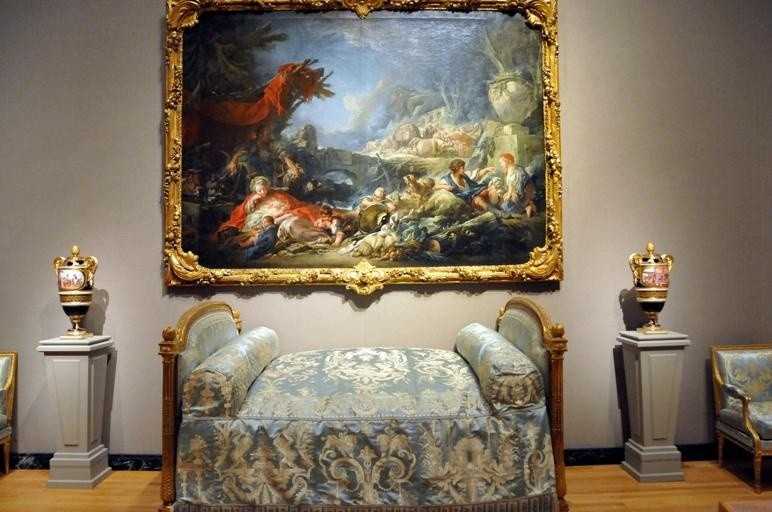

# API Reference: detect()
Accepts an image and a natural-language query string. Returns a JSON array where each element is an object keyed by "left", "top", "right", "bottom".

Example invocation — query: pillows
[
  {"left": 181, "top": 326, "right": 280, "bottom": 419},
  {"left": 455, "top": 321, "right": 546, "bottom": 414}
]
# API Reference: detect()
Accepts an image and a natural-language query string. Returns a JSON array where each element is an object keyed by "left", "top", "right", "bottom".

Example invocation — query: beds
[{"left": 159, "top": 297, "right": 569, "bottom": 512}]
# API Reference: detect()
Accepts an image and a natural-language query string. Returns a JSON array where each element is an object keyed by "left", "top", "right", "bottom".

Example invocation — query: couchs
[
  {"left": 0, "top": 352, "right": 18, "bottom": 475},
  {"left": 709, "top": 344, "right": 772, "bottom": 494}
]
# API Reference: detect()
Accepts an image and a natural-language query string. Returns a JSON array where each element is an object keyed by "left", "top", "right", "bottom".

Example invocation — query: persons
[
  {"left": 238, "top": 216, "right": 277, "bottom": 260},
  {"left": 354, "top": 187, "right": 396, "bottom": 215},
  {"left": 215, "top": 176, "right": 351, "bottom": 247},
  {"left": 314, "top": 204, "right": 345, "bottom": 247},
  {"left": 499, "top": 153, "right": 537, "bottom": 218},
  {"left": 432, "top": 159, "right": 512, "bottom": 219}
]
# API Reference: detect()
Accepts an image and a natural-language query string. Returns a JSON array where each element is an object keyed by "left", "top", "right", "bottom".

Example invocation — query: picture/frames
[{"left": 162, "top": 0, "right": 564, "bottom": 296}]
